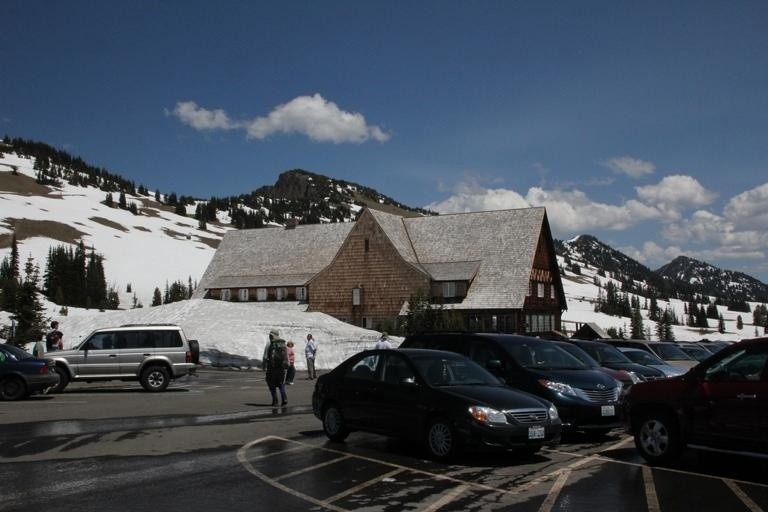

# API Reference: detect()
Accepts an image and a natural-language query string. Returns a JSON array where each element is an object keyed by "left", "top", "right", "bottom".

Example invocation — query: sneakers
[
  {"left": 305, "top": 377, "right": 313, "bottom": 380},
  {"left": 271, "top": 400, "right": 278, "bottom": 406},
  {"left": 289, "top": 382, "right": 294, "bottom": 385},
  {"left": 281, "top": 399, "right": 288, "bottom": 407}
]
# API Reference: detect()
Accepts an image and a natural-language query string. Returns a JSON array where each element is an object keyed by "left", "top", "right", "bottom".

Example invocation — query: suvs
[
  {"left": 616, "top": 335, "right": 768, "bottom": 466},
  {"left": 34, "top": 323, "right": 203, "bottom": 395}
]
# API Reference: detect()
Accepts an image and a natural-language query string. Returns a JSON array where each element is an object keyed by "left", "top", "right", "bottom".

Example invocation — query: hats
[
  {"left": 382, "top": 332, "right": 388, "bottom": 339},
  {"left": 264, "top": 328, "right": 282, "bottom": 340}
]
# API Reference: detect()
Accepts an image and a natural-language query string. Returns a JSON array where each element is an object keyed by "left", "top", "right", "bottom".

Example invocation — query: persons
[
  {"left": 46, "top": 321, "right": 64, "bottom": 352},
  {"left": 373, "top": 331, "right": 391, "bottom": 363},
  {"left": 286, "top": 341, "right": 296, "bottom": 385},
  {"left": 33, "top": 334, "right": 49, "bottom": 395},
  {"left": 305, "top": 334, "right": 317, "bottom": 380},
  {"left": 261, "top": 329, "right": 288, "bottom": 405}
]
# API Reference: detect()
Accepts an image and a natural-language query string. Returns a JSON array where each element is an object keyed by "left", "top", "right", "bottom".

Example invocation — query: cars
[
  {"left": 0, "top": 342, "right": 63, "bottom": 402},
  {"left": 519, "top": 326, "right": 736, "bottom": 408},
  {"left": 311, "top": 344, "right": 564, "bottom": 467}
]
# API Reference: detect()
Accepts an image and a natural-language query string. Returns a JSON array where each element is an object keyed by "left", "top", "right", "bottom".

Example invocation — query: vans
[{"left": 394, "top": 327, "right": 625, "bottom": 446}]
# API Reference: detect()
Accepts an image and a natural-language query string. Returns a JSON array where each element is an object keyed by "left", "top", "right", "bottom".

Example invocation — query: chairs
[{"left": 355, "top": 365, "right": 370, "bottom": 379}]
